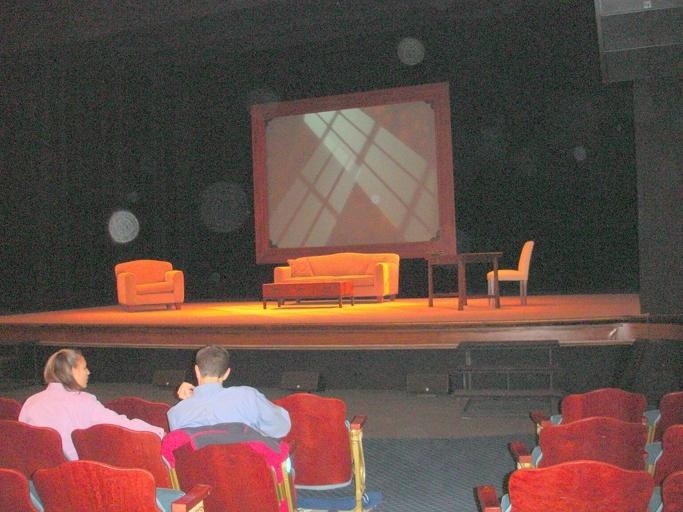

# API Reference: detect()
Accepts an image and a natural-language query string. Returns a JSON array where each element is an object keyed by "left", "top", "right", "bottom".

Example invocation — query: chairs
[
  {"left": 487, "top": 241, "right": 535, "bottom": 308},
  {"left": 115, "top": 260, "right": 185, "bottom": 312},
  {"left": 477, "top": 387, "right": 683, "bottom": 512}
]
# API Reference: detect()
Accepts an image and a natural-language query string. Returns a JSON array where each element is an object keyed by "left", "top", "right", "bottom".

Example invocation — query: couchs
[{"left": 274, "top": 252, "right": 400, "bottom": 304}]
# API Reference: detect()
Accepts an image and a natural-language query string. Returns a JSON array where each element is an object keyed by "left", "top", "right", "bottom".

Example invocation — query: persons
[
  {"left": 18, "top": 349, "right": 167, "bottom": 461},
  {"left": 166, "top": 344, "right": 291, "bottom": 439}
]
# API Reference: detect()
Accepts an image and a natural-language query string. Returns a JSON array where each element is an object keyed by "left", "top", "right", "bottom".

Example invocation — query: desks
[{"left": 428, "top": 252, "right": 502, "bottom": 310}]
